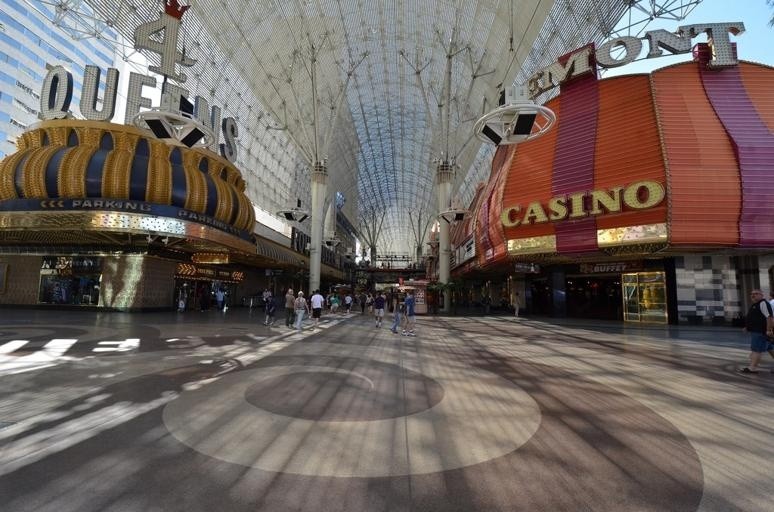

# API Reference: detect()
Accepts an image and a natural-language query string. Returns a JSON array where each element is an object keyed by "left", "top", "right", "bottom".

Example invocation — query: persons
[
  {"left": 740, "top": 289, "right": 774, "bottom": 374},
  {"left": 513, "top": 291, "right": 521, "bottom": 317},
  {"left": 198, "top": 287, "right": 225, "bottom": 312},
  {"left": 178, "top": 283, "right": 189, "bottom": 312},
  {"left": 262, "top": 287, "right": 416, "bottom": 336}
]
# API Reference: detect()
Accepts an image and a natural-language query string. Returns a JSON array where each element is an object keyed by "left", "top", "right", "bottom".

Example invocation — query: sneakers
[{"left": 372, "top": 324, "right": 416, "bottom": 337}]
